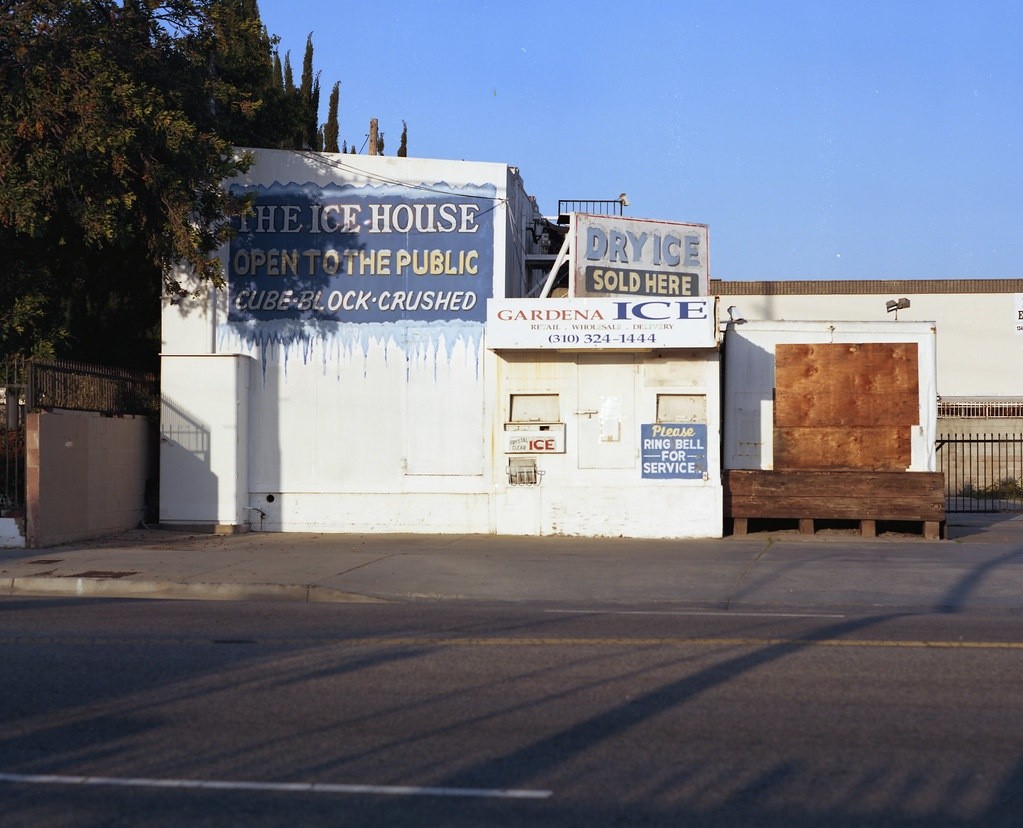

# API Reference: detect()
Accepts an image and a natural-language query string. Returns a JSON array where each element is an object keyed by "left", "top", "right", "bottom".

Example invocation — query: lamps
[
  {"left": 885, "top": 298, "right": 911, "bottom": 320},
  {"left": 618, "top": 193, "right": 631, "bottom": 207}
]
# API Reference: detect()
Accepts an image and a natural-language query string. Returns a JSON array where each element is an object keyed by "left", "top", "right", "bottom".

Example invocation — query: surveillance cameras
[
  {"left": 728, "top": 306, "right": 743, "bottom": 322},
  {"left": 620, "top": 193, "right": 631, "bottom": 207}
]
